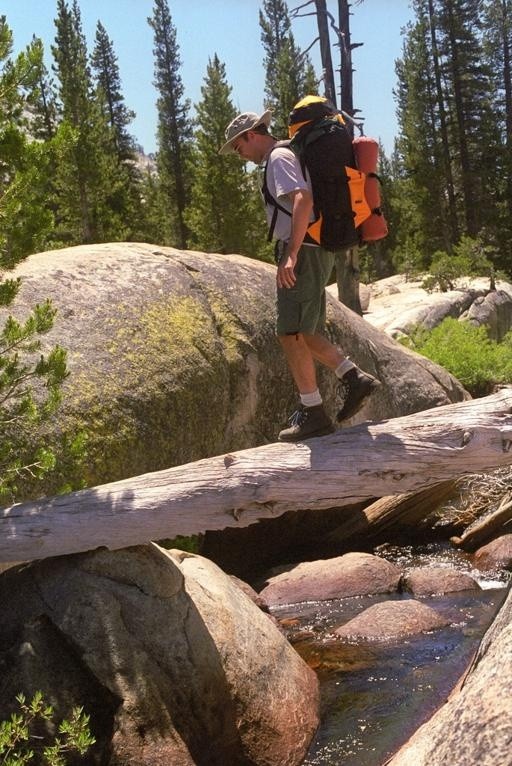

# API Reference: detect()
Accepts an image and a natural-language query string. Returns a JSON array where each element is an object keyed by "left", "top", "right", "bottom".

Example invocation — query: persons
[{"left": 218, "top": 95, "right": 389, "bottom": 442}]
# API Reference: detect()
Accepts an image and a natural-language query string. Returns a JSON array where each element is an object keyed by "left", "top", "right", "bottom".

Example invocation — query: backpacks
[{"left": 285, "top": 94, "right": 371, "bottom": 252}]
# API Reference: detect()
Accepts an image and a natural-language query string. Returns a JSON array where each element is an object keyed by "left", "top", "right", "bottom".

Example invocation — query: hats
[{"left": 218, "top": 110, "right": 272, "bottom": 157}]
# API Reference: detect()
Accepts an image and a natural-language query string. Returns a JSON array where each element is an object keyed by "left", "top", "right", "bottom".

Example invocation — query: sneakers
[
  {"left": 334, "top": 367, "right": 381, "bottom": 424},
  {"left": 276, "top": 402, "right": 336, "bottom": 443}
]
